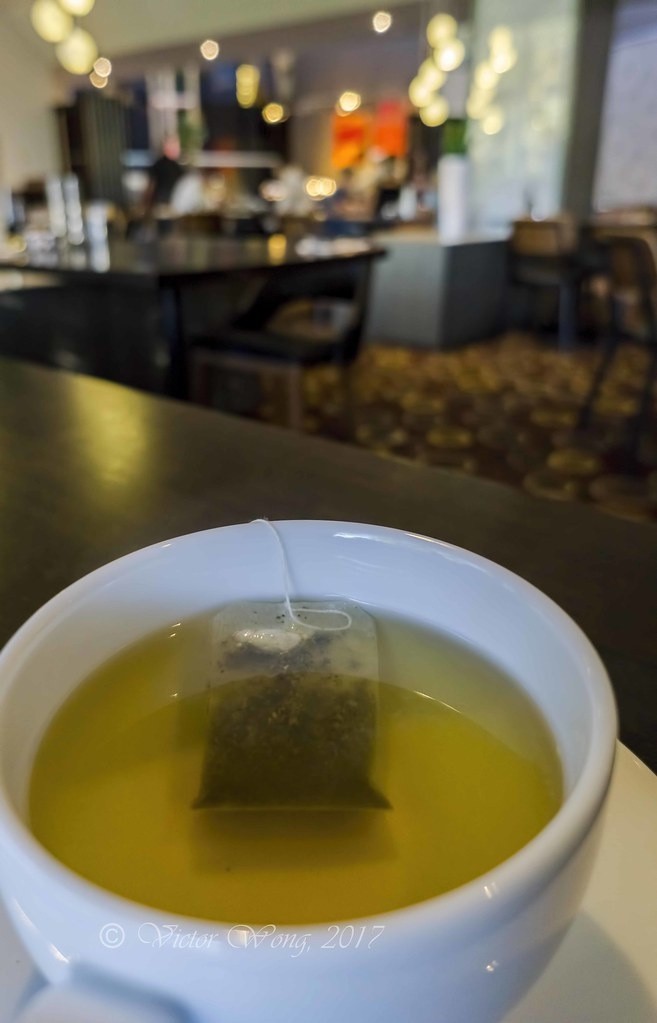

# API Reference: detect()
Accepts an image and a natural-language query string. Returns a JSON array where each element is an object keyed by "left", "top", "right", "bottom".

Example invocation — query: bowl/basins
[{"left": 0, "top": 516, "right": 619, "bottom": 1022}]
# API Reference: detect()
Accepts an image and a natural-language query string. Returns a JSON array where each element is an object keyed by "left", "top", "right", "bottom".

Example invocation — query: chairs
[{"left": 570, "top": 227, "right": 657, "bottom": 488}]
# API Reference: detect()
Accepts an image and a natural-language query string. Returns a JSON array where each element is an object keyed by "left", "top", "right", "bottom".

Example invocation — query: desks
[{"left": 366, "top": 217, "right": 513, "bottom": 350}]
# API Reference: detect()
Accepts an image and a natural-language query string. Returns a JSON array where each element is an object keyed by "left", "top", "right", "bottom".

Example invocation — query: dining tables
[
  {"left": 0, "top": 221, "right": 385, "bottom": 438},
  {"left": 0, "top": 353, "right": 657, "bottom": 1020}
]
